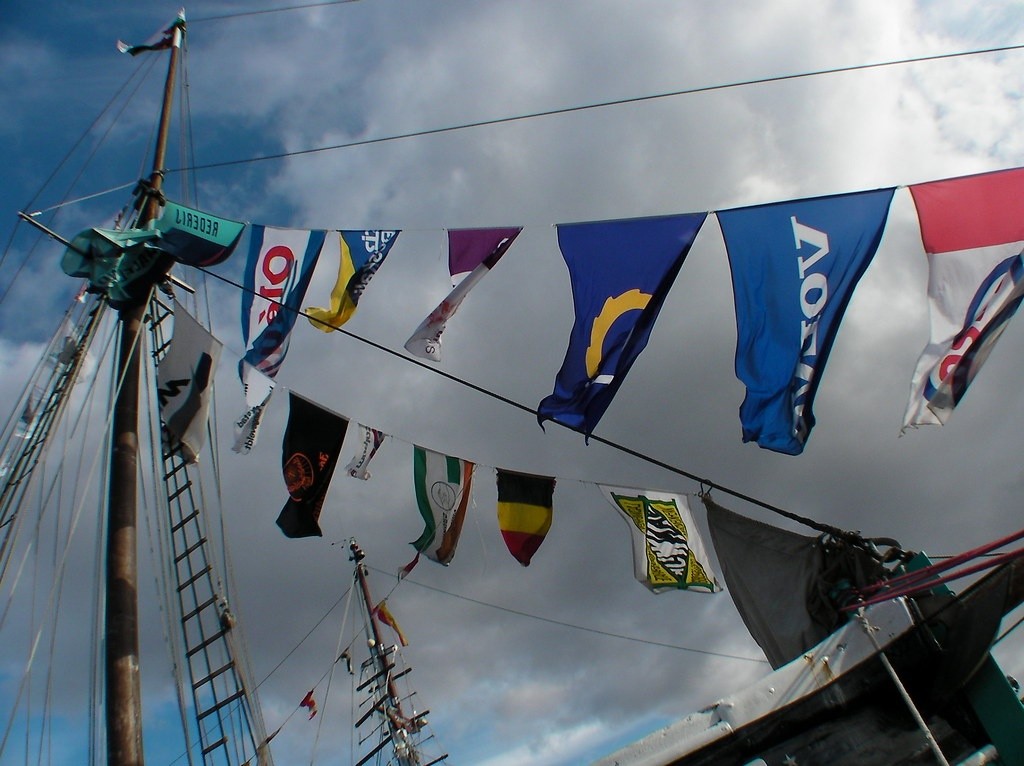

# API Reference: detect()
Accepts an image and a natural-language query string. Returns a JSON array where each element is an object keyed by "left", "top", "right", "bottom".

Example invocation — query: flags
[
  {"left": 408, "top": 445, "right": 474, "bottom": 567},
  {"left": 900, "top": 167, "right": 1024, "bottom": 433},
  {"left": 599, "top": 484, "right": 722, "bottom": 595},
  {"left": 276, "top": 392, "right": 348, "bottom": 538},
  {"left": 405, "top": 227, "right": 524, "bottom": 361},
  {"left": 345, "top": 425, "right": 385, "bottom": 480},
  {"left": 378, "top": 604, "right": 408, "bottom": 646},
  {"left": 240, "top": 225, "right": 325, "bottom": 376},
  {"left": 232, "top": 360, "right": 277, "bottom": 454},
  {"left": 716, "top": 187, "right": 896, "bottom": 456},
  {"left": 61, "top": 202, "right": 246, "bottom": 310},
  {"left": 495, "top": 468, "right": 557, "bottom": 569},
  {"left": 301, "top": 693, "right": 319, "bottom": 719},
  {"left": 305, "top": 230, "right": 401, "bottom": 333},
  {"left": 158, "top": 299, "right": 221, "bottom": 460},
  {"left": 536, "top": 211, "right": 707, "bottom": 446}
]
